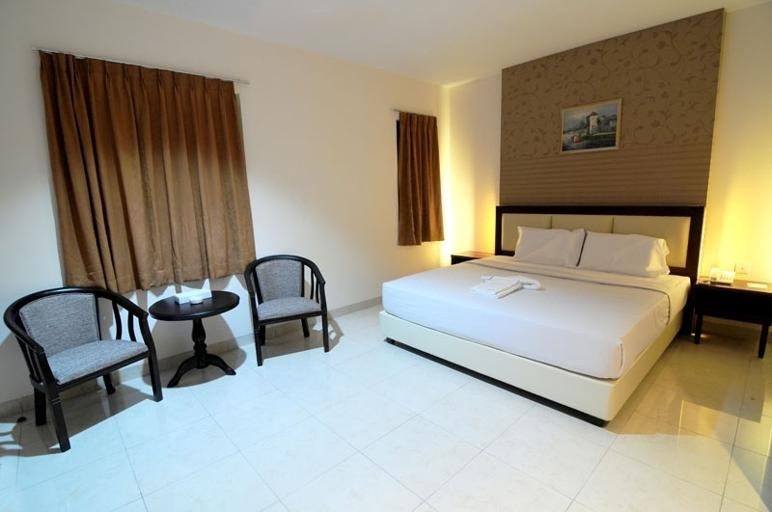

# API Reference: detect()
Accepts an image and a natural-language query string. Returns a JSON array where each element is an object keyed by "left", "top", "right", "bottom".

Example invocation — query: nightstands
[
  {"left": 451, "top": 251, "right": 494, "bottom": 265},
  {"left": 694, "top": 278, "right": 772, "bottom": 359}
]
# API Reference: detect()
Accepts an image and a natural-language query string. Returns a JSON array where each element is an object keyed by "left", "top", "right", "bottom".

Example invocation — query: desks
[{"left": 148, "top": 290, "right": 240, "bottom": 388}]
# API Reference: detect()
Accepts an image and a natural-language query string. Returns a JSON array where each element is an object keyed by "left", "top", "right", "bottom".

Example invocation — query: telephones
[{"left": 710, "top": 268, "right": 736, "bottom": 285}]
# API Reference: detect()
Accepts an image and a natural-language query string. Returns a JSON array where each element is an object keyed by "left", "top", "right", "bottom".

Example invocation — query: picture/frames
[{"left": 559, "top": 96, "right": 622, "bottom": 156}]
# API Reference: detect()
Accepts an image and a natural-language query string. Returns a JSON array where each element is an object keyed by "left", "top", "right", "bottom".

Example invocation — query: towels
[
  {"left": 480, "top": 274, "right": 542, "bottom": 290},
  {"left": 471, "top": 278, "right": 523, "bottom": 300}
]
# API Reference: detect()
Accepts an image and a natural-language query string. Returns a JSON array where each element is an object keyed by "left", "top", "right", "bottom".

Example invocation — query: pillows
[
  {"left": 577, "top": 230, "right": 671, "bottom": 278},
  {"left": 512, "top": 226, "right": 586, "bottom": 268}
]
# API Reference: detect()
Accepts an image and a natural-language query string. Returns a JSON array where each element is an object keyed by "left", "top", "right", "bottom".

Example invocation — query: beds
[{"left": 378, "top": 205, "right": 704, "bottom": 427}]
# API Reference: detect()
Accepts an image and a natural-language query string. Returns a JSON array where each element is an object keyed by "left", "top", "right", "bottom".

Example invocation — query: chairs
[
  {"left": 3, "top": 287, "right": 163, "bottom": 452},
  {"left": 244, "top": 254, "right": 329, "bottom": 366}
]
